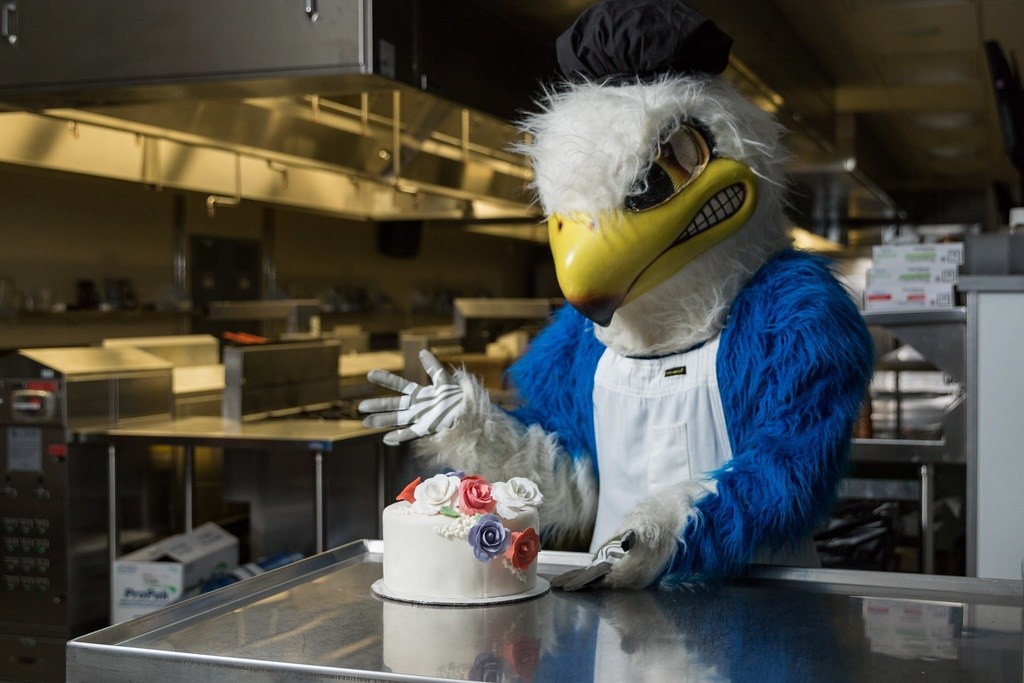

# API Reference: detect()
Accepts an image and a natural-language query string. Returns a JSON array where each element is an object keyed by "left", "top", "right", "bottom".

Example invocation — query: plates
[{"left": 371, "top": 576, "right": 552, "bottom": 605}]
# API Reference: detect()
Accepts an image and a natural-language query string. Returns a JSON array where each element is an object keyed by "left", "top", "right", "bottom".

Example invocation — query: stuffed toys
[{"left": 359, "top": 0, "right": 875, "bottom": 592}]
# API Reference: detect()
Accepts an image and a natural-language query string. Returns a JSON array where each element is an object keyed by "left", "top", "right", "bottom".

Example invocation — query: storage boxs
[
  {"left": 858, "top": 592, "right": 965, "bottom": 662},
  {"left": 110, "top": 523, "right": 237, "bottom": 626},
  {"left": 863, "top": 241, "right": 963, "bottom": 309}
]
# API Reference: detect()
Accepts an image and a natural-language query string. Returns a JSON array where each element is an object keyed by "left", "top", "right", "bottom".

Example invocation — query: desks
[
  {"left": 67, "top": 540, "right": 1024, "bottom": 683},
  {"left": 95, "top": 415, "right": 399, "bottom": 555}
]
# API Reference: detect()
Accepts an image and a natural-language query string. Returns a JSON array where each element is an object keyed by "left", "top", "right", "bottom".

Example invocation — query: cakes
[{"left": 383, "top": 470, "right": 543, "bottom": 598}]
